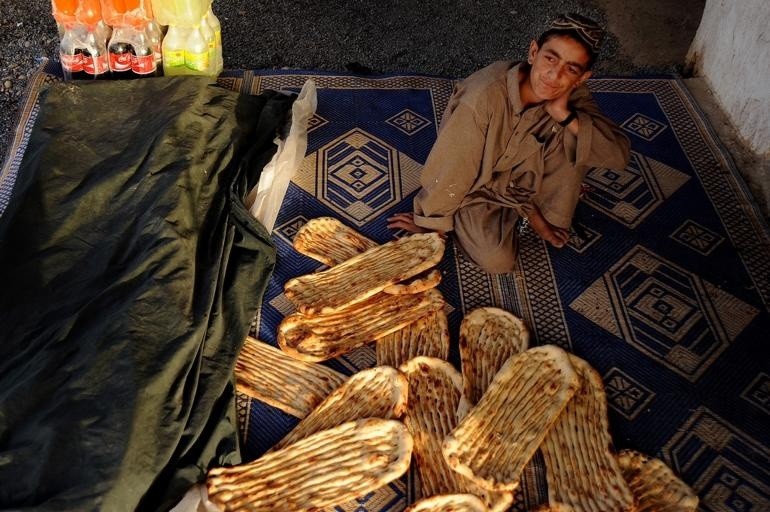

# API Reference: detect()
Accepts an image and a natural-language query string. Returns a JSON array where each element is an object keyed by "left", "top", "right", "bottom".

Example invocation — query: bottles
[{"left": 50, "top": 0, "right": 227, "bottom": 80}]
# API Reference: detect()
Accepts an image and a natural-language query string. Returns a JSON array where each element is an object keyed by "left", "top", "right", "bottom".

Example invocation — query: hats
[{"left": 550, "top": 12, "right": 605, "bottom": 54}]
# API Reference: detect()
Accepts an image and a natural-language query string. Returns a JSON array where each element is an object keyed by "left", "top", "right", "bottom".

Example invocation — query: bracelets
[{"left": 558, "top": 112, "right": 576, "bottom": 126}]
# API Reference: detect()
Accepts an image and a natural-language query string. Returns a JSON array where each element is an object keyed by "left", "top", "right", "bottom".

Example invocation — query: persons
[{"left": 386, "top": 14, "right": 631, "bottom": 275}]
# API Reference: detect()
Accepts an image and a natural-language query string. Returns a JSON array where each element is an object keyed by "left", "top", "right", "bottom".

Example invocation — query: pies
[{"left": 203, "top": 216, "right": 700, "bottom": 511}]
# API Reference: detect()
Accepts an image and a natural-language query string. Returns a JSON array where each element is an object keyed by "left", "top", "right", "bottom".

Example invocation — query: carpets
[{"left": 0, "top": 54, "right": 769, "bottom": 511}]
